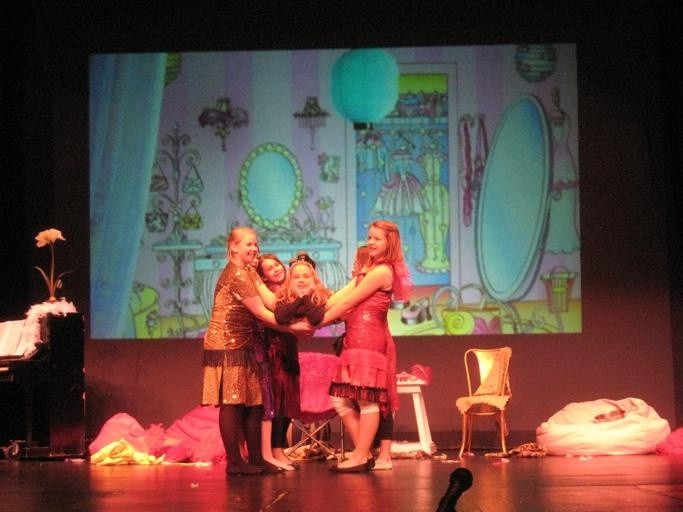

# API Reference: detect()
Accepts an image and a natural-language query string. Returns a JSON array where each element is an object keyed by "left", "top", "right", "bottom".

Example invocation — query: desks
[{"left": 191, "top": 240, "right": 347, "bottom": 330}]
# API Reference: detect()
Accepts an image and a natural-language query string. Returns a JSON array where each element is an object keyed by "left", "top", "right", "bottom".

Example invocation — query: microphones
[{"left": 437, "top": 468, "right": 472, "bottom": 512}]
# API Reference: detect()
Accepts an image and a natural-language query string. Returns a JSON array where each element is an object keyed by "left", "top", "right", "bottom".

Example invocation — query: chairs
[{"left": 456, "top": 347, "right": 513, "bottom": 459}]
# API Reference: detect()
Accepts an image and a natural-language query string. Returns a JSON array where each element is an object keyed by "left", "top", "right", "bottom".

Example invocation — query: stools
[{"left": 391, "top": 380, "right": 437, "bottom": 459}]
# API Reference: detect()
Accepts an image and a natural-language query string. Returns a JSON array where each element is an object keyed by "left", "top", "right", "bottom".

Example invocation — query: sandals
[
  {"left": 227, "top": 462, "right": 299, "bottom": 475},
  {"left": 329, "top": 457, "right": 392, "bottom": 472}
]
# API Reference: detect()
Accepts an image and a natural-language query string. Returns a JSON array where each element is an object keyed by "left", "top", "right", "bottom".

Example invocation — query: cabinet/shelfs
[{"left": 145, "top": 121, "right": 204, "bottom": 339}]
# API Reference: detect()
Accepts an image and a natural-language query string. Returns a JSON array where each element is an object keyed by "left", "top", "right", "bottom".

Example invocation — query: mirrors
[
  {"left": 475, "top": 92, "right": 554, "bottom": 307},
  {"left": 239, "top": 141, "right": 304, "bottom": 232}
]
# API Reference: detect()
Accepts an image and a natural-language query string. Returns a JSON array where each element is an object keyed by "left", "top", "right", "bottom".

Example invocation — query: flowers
[{"left": 34, "top": 228, "right": 74, "bottom": 302}]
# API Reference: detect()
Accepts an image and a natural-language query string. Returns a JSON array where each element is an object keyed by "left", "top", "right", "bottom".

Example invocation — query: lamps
[{"left": 330, "top": 48, "right": 399, "bottom": 130}]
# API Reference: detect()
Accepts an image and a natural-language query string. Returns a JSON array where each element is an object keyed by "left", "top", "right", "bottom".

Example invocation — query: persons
[{"left": 196, "top": 220, "right": 413, "bottom": 475}]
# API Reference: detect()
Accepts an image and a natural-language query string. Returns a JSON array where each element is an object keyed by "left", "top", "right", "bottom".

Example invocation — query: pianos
[{"left": 0, "top": 313, "right": 86, "bottom": 461}]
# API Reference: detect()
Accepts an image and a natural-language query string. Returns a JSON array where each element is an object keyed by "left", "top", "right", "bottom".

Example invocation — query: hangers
[{"left": 356, "top": 128, "right": 445, "bottom": 152}]
